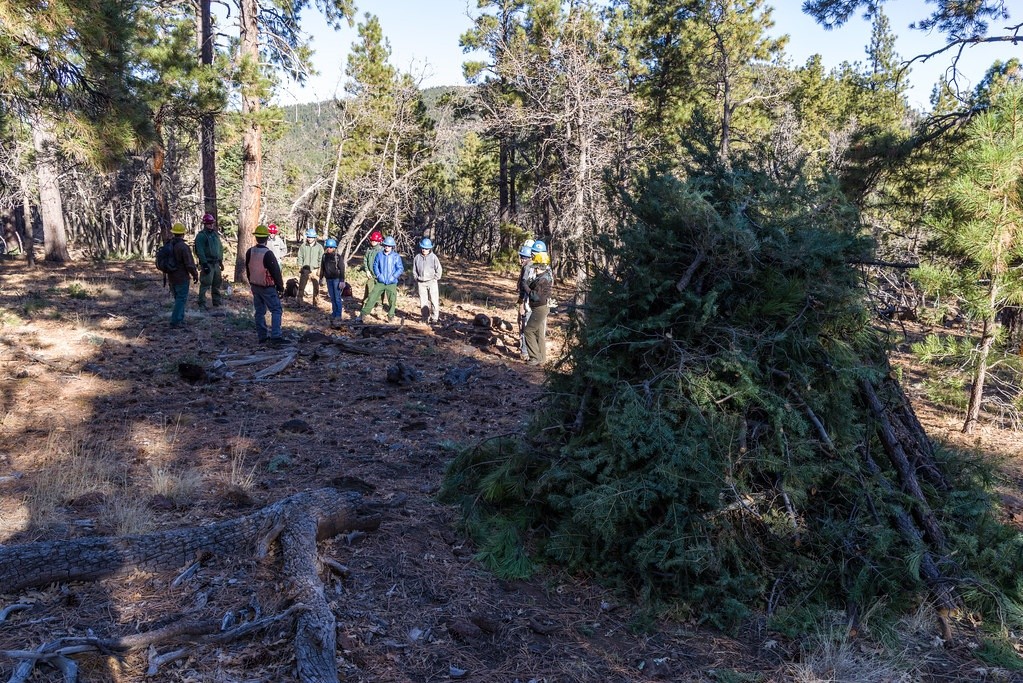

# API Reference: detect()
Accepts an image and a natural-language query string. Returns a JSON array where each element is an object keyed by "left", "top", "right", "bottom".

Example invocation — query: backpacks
[{"left": 156, "top": 239, "right": 185, "bottom": 273}]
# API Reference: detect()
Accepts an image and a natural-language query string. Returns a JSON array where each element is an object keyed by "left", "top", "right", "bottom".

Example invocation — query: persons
[
  {"left": 243, "top": 225, "right": 292, "bottom": 344},
  {"left": 412, "top": 239, "right": 442, "bottom": 321},
  {"left": 297, "top": 228, "right": 324, "bottom": 307},
  {"left": 360, "top": 236, "right": 403, "bottom": 322},
  {"left": 169, "top": 223, "right": 198, "bottom": 329},
  {"left": 364, "top": 231, "right": 385, "bottom": 313},
  {"left": 266, "top": 223, "right": 288, "bottom": 274},
  {"left": 318, "top": 239, "right": 347, "bottom": 321},
  {"left": 195, "top": 214, "right": 227, "bottom": 312},
  {"left": 516, "top": 238, "right": 553, "bottom": 365}
]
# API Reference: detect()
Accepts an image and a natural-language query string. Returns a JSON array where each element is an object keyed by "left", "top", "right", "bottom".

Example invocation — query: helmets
[
  {"left": 201, "top": 214, "right": 216, "bottom": 223},
  {"left": 252, "top": 225, "right": 270, "bottom": 237},
  {"left": 420, "top": 239, "right": 433, "bottom": 248},
  {"left": 268, "top": 224, "right": 278, "bottom": 233},
  {"left": 304, "top": 229, "right": 317, "bottom": 238},
  {"left": 518, "top": 246, "right": 532, "bottom": 257},
  {"left": 170, "top": 223, "right": 186, "bottom": 234},
  {"left": 325, "top": 239, "right": 338, "bottom": 248},
  {"left": 532, "top": 240, "right": 547, "bottom": 252},
  {"left": 382, "top": 237, "right": 396, "bottom": 246},
  {"left": 369, "top": 232, "right": 383, "bottom": 241},
  {"left": 531, "top": 253, "right": 550, "bottom": 264}
]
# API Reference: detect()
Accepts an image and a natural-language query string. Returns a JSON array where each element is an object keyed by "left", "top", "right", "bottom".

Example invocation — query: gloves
[{"left": 202, "top": 263, "right": 211, "bottom": 274}]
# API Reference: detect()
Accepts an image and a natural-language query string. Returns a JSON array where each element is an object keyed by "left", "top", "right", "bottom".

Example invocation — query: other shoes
[
  {"left": 259, "top": 336, "right": 270, "bottom": 343},
  {"left": 270, "top": 338, "right": 291, "bottom": 345},
  {"left": 170, "top": 322, "right": 187, "bottom": 329},
  {"left": 352, "top": 319, "right": 363, "bottom": 325},
  {"left": 527, "top": 360, "right": 543, "bottom": 365}
]
